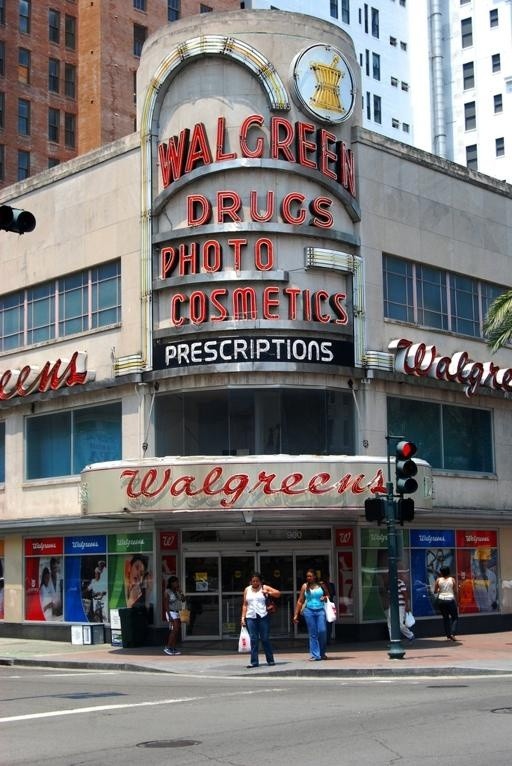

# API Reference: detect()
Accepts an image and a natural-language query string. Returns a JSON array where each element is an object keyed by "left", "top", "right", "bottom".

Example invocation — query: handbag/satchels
[
  {"left": 300, "top": 583, "right": 307, "bottom": 616},
  {"left": 179, "top": 610, "right": 191, "bottom": 623},
  {"left": 262, "top": 585, "right": 277, "bottom": 615}
]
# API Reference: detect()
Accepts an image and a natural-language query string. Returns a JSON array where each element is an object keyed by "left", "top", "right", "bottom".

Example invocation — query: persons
[
  {"left": 239, "top": 573, "right": 281, "bottom": 669},
  {"left": 85, "top": 567, "right": 102, "bottom": 615},
  {"left": 98, "top": 561, "right": 108, "bottom": 621},
  {"left": 48, "top": 557, "right": 64, "bottom": 622},
  {"left": 122, "top": 554, "right": 154, "bottom": 610},
  {"left": 385, "top": 568, "right": 415, "bottom": 643},
  {"left": 162, "top": 574, "right": 187, "bottom": 655},
  {"left": 317, "top": 570, "right": 337, "bottom": 646},
  {"left": 432, "top": 566, "right": 459, "bottom": 643},
  {"left": 292, "top": 569, "right": 331, "bottom": 661},
  {"left": 471, "top": 558, "right": 499, "bottom": 611},
  {"left": 39, "top": 566, "right": 57, "bottom": 621}
]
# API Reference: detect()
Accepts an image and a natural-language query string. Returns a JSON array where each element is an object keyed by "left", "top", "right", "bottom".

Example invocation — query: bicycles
[{"left": 92, "top": 593, "right": 106, "bottom": 623}]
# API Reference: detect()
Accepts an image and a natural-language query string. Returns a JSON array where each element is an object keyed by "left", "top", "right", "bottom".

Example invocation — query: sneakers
[
  {"left": 162, "top": 646, "right": 175, "bottom": 656},
  {"left": 173, "top": 648, "right": 181, "bottom": 655}
]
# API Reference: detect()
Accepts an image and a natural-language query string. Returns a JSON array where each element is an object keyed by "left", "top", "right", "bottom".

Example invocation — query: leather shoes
[
  {"left": 268, "top": 661, "right": 275, "bottom": 665},
  {"left": 247, "top": 664, "right": 258, "bottom": 667}
]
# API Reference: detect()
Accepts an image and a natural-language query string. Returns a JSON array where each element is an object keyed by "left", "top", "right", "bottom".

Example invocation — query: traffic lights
[
  {"left": 395, "top": 440, "right": 419, "bottom": 494},
  {"left": 0, "top": 200, "right": 40, "bottom": 235},
  {"left": 393, "top": 498, "right": 415, "bottom": 523},
  {"left": 363, "top": 498, "right": 385, "bottom": 521}
]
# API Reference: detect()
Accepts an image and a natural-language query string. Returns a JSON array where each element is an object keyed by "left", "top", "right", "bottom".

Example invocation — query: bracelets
[{"left": 179, "top": 592, "right": 183, "bottom": 595}]
